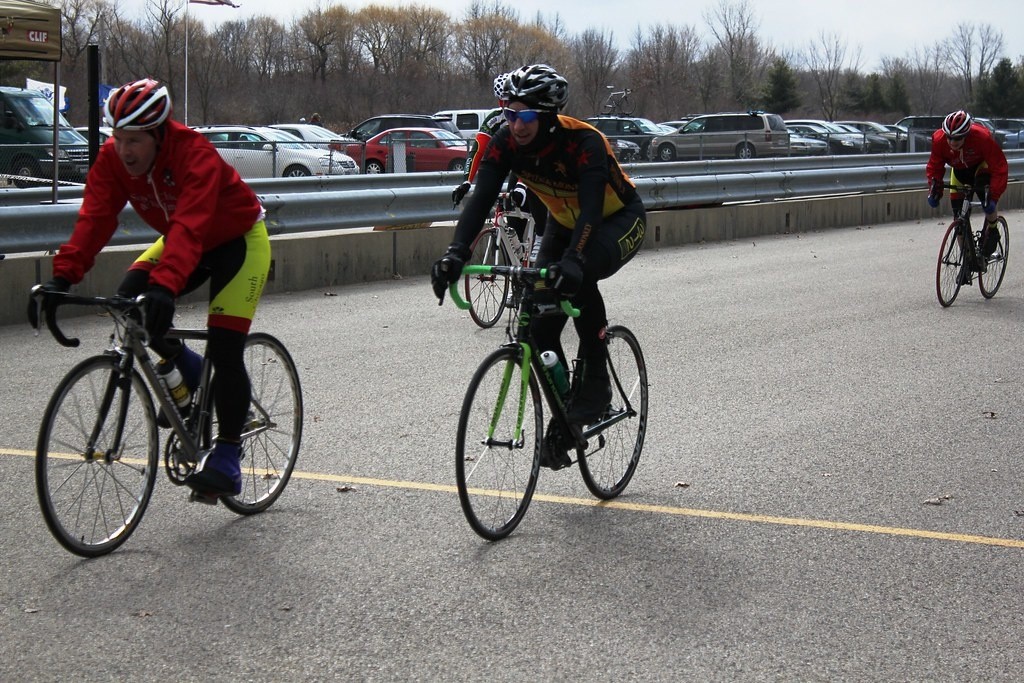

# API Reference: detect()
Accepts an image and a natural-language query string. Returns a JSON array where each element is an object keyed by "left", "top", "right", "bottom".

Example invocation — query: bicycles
[
  {"left": 26, "top": 284, "right": 304, "bottom": 558},
  {"left": 928, "top": 176, "right": 1009, "bottom": 307},
  {"left": 598, "top": 84, "right": 637, "bottom": 115},
  {"left": 436, "top": 255, "right": 648, "bottom": 541},
  {"left": 450, "top": 185, "right": 542, "bottom": 328}
]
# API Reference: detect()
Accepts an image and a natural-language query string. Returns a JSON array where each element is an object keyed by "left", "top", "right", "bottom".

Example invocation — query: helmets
[
  {"left": 504, "top": 63, "right": 570, "bottom": 113},
  {"left": 103, "top": 77, "right": 173, "bottom": 133},
  {"left": 493, "top": 73, "right": 512, "bottom": 101},
  {"left": 942, "top": 110, "right": 971, "bottom": 138}
]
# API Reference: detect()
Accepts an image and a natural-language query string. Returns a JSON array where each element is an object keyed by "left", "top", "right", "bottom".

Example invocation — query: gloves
[
  {"left": 984, "top": 201, "right": 996, "bottom": 215},
  {"left": 450, "top": 181, "right": 471, "bottom": 206},
  {"left": 39, "top": 276, "right": 72, "bottom": 312},
  {"left": 507, "top": 181, "right": 528, "bottom": 207},
  {"left": 545, "top": 247, "right": 587, "bottom": 298},
  {"left": 431, "top": 242, "right": 473, "bottom": 299},
  {"left": 928, "top": 193, "right": 940, "bottom": 207},
  {"left": 120, "top": 283, "right": 176, "bottom": 346}
]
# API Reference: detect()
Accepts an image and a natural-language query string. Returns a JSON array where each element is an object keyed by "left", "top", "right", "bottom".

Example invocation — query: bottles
[
  {"left": 157, "top": 359, "right": 191, "bottom": 407},
  {"left": 506, "top": 227, "right": 523, "bottom": 259},
  {"left": 540, "top": 350, "right": 570, "bottom": 396}
]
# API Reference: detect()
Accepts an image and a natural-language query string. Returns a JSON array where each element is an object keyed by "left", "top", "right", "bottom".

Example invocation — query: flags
[{"left": 187, "top": 0, "right": 242, "bottom": 8}]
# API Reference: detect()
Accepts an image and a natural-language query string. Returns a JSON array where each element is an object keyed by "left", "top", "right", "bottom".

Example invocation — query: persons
[
  {"left": 432, "top": 62, "right": 646, "bottom": 471},
  {"left": 926, "top": 111, "right": 1009, "bottom": 285},
  {"left": 46, "top": 76, "right": 272, "bottom": 498}
]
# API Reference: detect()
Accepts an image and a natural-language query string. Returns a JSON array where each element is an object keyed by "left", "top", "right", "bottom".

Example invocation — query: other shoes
[
  {"left": 537, "top": 417, "right": 582, "bottom": 467},
  {"left": 565, "top": 370, "right": 612, "bottom": 421},
  {"left": 154, "top": 337, "right": 205, "bottom": 429},
  {"left": 979, "top": 227, "right": 1001, "bottom": 257},
  {"left": 502, "top": 295, "right": 522, "bottom": 308},
  {"left": 956, "top": 256, "right": 975, "bottom": 285},
  {"left": 184, "top": 437, "right": 242, "bottom": 497}
]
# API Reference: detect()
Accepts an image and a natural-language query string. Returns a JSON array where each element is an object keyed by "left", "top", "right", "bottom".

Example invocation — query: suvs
[
  {"left": 340, "top": 114, "right": 464, "bottom": 142},
  {"left": 993, "top": 118, "right": 1024, "bottom": 149},
  {"left": 583, "top": 116, "right": 666, "bottom": 161},
  {"left": 647, "top": 111, "right": 789, "bottom": 162},
  {"left": 893, "top": 114, "right": 997, "bottom": 143}
]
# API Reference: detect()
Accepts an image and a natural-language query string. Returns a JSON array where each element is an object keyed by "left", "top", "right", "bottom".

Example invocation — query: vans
[
  {"left": 0, "top": 87, "right": 89, "bottom": 189},
  {"left": 431, "top": 109, "right": 493, "bottom": 141}
]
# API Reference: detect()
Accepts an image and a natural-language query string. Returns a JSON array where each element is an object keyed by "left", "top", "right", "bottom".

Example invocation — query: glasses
[
  {"left": 501, "top": 108, "right": 550, "bottom": 124},
  {"left": 945, "top": 132, "right": 966, "bottom": 142}
]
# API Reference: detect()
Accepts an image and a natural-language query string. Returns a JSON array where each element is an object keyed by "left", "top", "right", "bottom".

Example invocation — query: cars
[
  {"left": 624, "top": 113, "right": 709, "bottom": 133},
  {"left": 186, "top": 124, "right": 360, "bottom": 178},
  {"left": 346, "top": 127, "right": 475, "bottom": 174},
  {"left": 216, "top": 122, "right": 359, "bottom": 156},
  {"left": 783, "top": 120, "right": 933, "bottom": 157},
  {"left": 74, "top": 125, "right": 114, "bottom": 144},
  {"left": 604, "top": 137, "right": 642, "bottom": 163}
]
